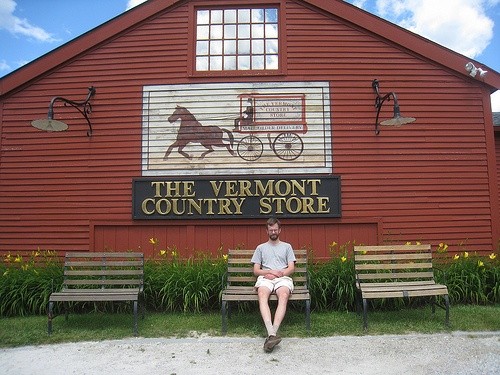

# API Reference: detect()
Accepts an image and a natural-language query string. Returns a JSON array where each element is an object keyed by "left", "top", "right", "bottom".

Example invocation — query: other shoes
[{"left": 263, "top": 335, "right": 283, "bottom": 353}]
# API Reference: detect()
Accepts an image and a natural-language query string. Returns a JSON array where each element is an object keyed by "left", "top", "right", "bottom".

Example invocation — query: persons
[{"left": 251, "top": 218, "right": 296, "bottom": 353}]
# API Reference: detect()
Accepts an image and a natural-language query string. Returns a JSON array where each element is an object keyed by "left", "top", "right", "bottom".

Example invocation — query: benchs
[
  {"left": 354, "top": 245, "right": 449, "bottom": 330},
  {"left": 222, "top": 249, "right": 311, "bottom": 331},
  {"left": 48, "top": 252, "right": 144, "bottom": 336}
]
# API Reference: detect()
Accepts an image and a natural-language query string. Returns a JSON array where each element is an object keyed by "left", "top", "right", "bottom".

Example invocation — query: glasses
[{"left": 269, "top": 229, "right": 280, "bottom": 232}]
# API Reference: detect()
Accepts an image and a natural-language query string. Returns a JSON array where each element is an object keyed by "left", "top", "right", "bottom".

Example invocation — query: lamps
[
  {"left": 31, "top": 86, "right": 95, "bottom": 136},
  {"left": 372, "top": 78, "right": 416, "bottom": 135}
]
